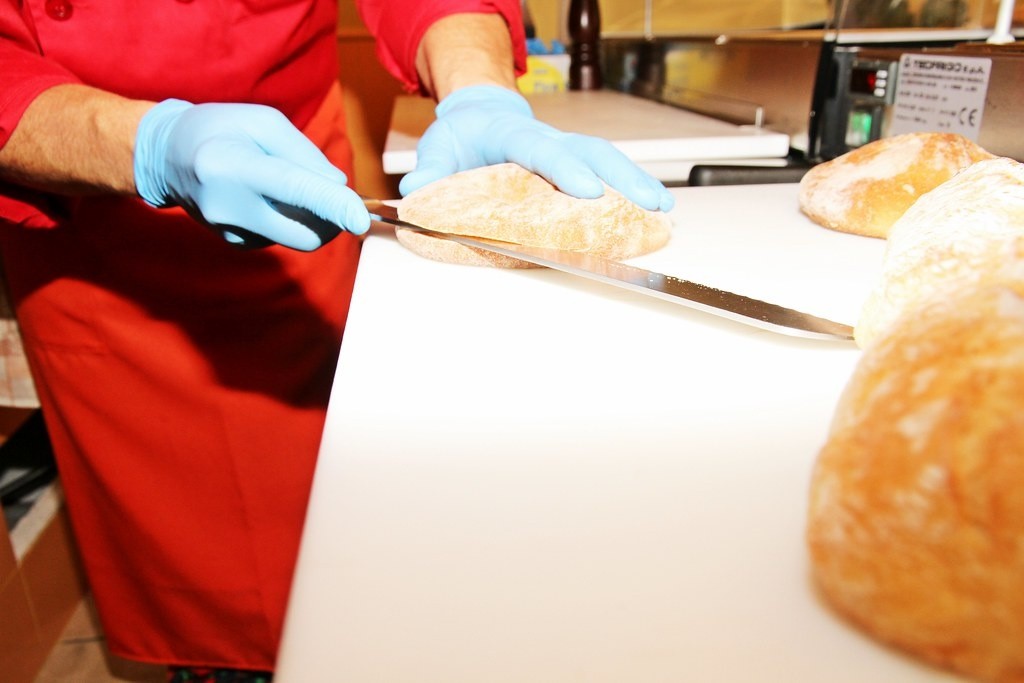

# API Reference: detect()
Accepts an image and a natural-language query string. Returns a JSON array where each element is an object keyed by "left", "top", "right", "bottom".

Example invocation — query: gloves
[
  {"left": 135, "top": 96, "right": 382, "bottom": 252},
  {"left": 398, "top": 85, "right": 678, "bottom": 215}
]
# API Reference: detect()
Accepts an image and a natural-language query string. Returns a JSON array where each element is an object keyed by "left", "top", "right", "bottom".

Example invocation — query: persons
[{"left": 0, "top": 0, "right": 675, "bottom": 671}]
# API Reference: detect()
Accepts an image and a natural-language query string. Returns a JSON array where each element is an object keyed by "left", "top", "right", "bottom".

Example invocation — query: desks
[{"left": 381, "top": 87, "right": 790, "bottom": 181}]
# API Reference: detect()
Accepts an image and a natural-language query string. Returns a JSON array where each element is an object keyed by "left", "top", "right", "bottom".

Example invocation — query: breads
[
  {"left": 798, "top": 130, "right": 1003, "bottom": 241},
  {"left": 393, "top": 162, "right": 670, "bottom": 269},
  {"left": 804, "top": 157, "right": 1024, "bottom": 683}
]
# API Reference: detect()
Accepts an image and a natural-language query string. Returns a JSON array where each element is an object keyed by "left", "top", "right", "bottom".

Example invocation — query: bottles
[{"left": 566, "top": 0, "right": 602, "bottom": 90}]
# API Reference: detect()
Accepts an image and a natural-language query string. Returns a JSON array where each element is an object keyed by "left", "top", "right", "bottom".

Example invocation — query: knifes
[{"left": 366, "top": 213, "right": 857, "bottom": 342}]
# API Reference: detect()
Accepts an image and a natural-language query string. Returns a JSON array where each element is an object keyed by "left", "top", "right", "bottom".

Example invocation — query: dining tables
[{"left": 271, "top": 183, "right": 1024, "bottom": 683}]
